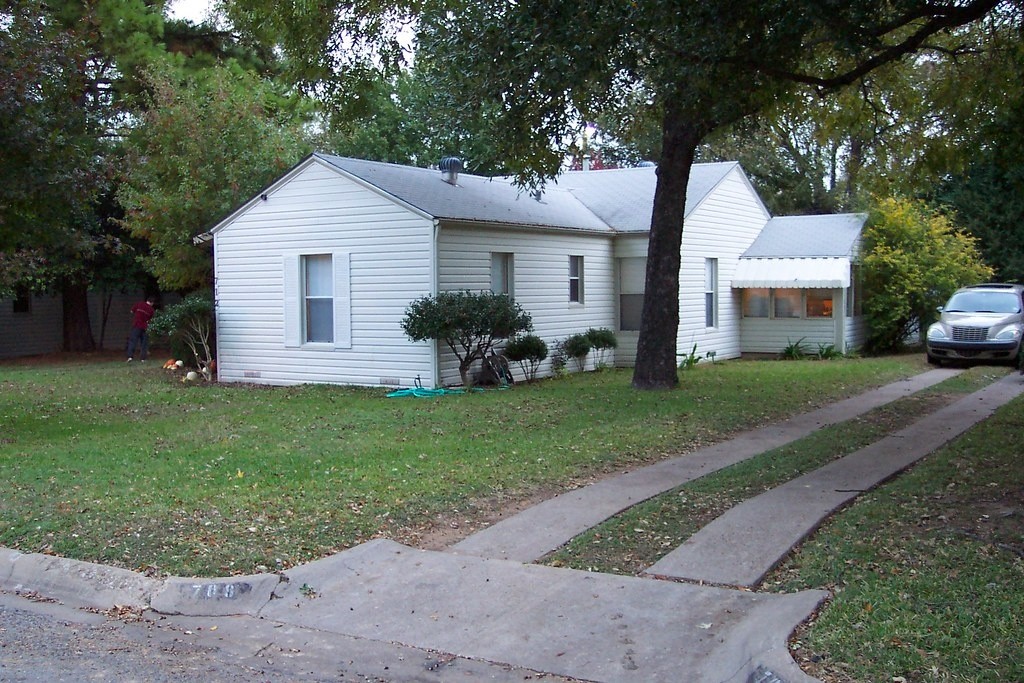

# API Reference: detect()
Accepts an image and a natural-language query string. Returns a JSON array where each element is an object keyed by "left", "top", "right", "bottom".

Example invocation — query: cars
[{"left": 926, "top": 284, "right": 1024, "bottom": 366}]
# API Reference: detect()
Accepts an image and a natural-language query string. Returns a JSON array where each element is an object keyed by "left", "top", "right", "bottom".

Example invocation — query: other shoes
[
  {"left": 141, "top": 360, "right": 145, "bottom": 363},
  {"left": 127, "top": 358, "right": 132, "bottom": 363}
]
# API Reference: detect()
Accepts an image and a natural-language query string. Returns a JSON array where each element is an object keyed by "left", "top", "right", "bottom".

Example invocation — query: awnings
[{"left": 730, "top": 258, "right": 851, "bottom": 289}]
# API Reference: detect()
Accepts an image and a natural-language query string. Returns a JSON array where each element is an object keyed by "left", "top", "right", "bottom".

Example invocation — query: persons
[{"left": 127, "top": 298, "right": 154, "bottom": 363}]
